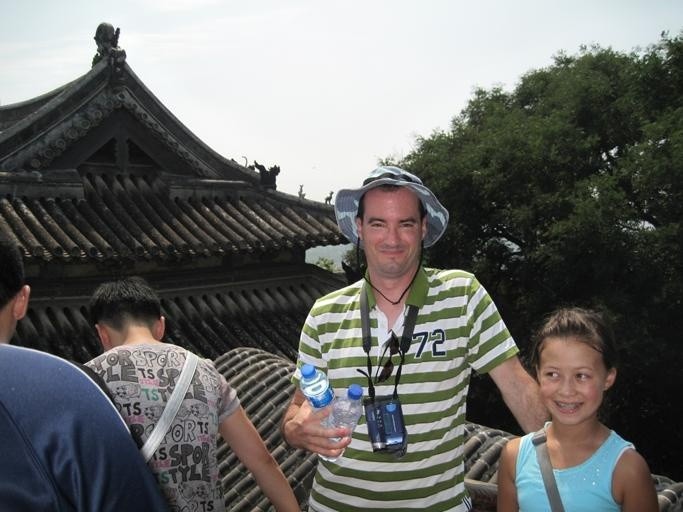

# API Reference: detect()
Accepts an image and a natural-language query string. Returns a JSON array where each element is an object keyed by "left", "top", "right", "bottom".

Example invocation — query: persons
[
  {"left": 0, "top": 212, "right": 175, "bottom": 509},
  {"left": 71, "top": 274, "right": 304, "bottom": 512},
  {"left": 277, "top": 165, "right": 554, "bottom": 511},
  {"left": 493, "top": 301, "right": 662, "bottom": 511}
]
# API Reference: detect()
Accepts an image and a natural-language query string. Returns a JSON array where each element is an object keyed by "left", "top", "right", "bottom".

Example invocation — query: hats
[{"left": 334, "top": 166, "right": 450, "bottom": 251}]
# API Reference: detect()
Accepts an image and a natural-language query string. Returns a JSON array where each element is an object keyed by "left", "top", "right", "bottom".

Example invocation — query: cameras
[{"left": 364, "top": 395, "right": 405, "bottom": 453}]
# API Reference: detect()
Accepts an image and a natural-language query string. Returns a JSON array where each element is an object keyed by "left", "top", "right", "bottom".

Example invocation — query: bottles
[
  {"left": 317, "top": 383, "right": 363, "bottom": 463},
  {"left": 300, "top": 363, "right": 339, "bottom": 429}
]
